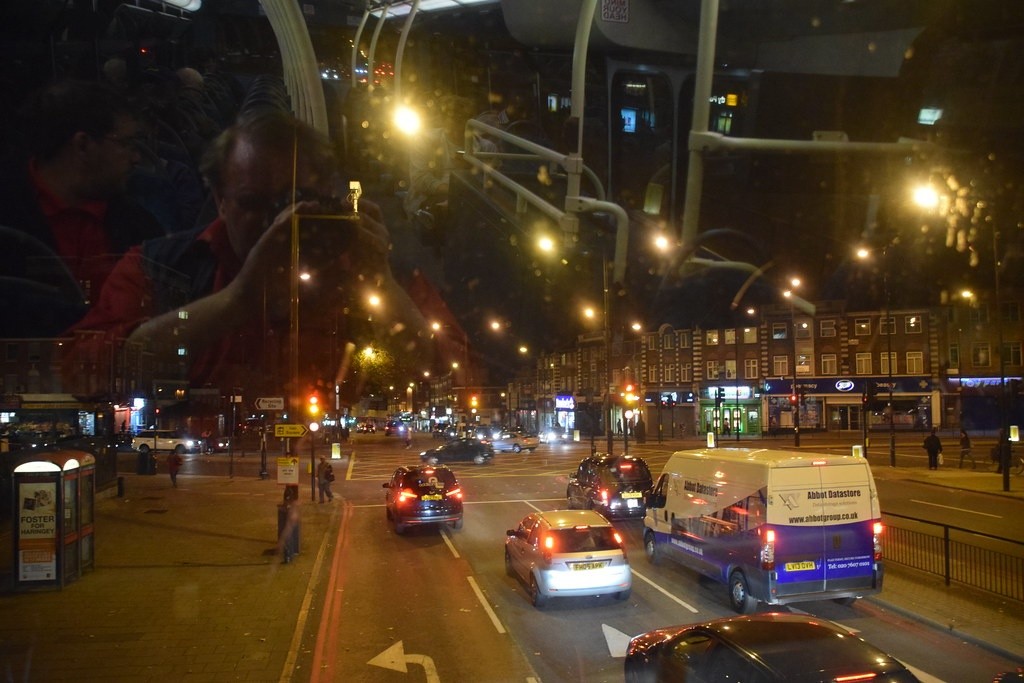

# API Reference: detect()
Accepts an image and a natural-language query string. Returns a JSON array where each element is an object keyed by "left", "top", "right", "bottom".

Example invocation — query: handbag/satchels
[
  {"left": 175, "top": 459, "right": 183, "bottom": 465},
  {"left": 937, "top": 454, "right": 943, "bottom": 465},
  {"left": 329, "top": 473, "right": 335, "bottom": 482}
]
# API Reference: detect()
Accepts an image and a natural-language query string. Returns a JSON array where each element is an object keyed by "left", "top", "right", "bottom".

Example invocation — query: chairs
[{"left": 0, "top": 43, "right": 783, "bottom": 368}]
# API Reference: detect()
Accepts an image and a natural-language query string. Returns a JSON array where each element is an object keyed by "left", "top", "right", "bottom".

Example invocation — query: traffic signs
[
  {"left": 275, "top": 424, "right": 307, "bottom": 438},
  {"left": 277, "top": 458, "right": 299, "bottom": 486},
  {"left": 255, "top": 399, "right": 285, "bottom": 411}
]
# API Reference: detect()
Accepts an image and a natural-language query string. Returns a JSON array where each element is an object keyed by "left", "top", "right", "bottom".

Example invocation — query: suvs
[
  {"left": 131, "top": 430, "right": 200, "bottom": 454},
  {"left": 380, "top": 466, "right": 465, "bottom": 535},
  {"left": 566, "top": 456, "right": 654, "bottom": 517}
]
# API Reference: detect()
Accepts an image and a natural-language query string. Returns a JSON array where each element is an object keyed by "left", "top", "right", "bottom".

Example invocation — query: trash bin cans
[
  {"left": 276, "top": 502, "right": 300, "bottom": 556},
  {"left": 138, "top": 452, "right": 158, "bottom": 475}
]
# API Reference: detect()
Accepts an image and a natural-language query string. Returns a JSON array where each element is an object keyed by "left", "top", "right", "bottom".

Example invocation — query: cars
[
  {"left": 432, "top": 423, "right": 494, "bottom": 441},
  {"left": 385, "top": 420, "right": 404, "bottom": 436},
  {"left": 201, "top": 429, "right": 231, "bottom": 453},
  {"left": 625, "top": 612, "right": 950, "bottom": 683},
  {"left": 420, "top": 438, "right": 494, "bottom": 465},
  {"left": 538, "top": 427, "right": 568, "bottom": 444},
  {"left": 491, "top": 426, "right": 540, "bottom": 453},
  {"left": 504, "top": 508, "right": 632, "bottom": 609},
  {"left": 355, "top": 424, "right": 377, "bottom": 433}
]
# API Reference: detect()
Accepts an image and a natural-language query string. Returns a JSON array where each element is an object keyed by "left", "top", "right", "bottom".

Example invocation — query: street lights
[
  {"left": 912, "top": 184, "right": 1010, "bottom": 492},
  {"left": 858, "top": 248, "right": 898, "bottom": 466},
  {"left": 332, "top": 290, "right": 380, "bottom": 397},
  {"left": 783, "top": 278, "right": 804, "bottom": 449},
  {"left": 732, "top": 305, "right": 756, "bottom": 441}
]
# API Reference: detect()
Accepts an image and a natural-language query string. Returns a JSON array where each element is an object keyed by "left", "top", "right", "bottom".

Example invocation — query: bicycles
[{"left": 982, "top": 447, "right": 1023, "bottom": 476}]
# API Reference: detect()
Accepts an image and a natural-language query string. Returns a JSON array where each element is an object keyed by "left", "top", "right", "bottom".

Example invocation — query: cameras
[{"left": 290, "top": 214, "right": 359, "bottom": 258}]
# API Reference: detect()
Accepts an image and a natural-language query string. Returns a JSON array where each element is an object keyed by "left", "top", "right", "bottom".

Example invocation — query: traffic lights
[
  {"left": 625, "top": 385, "right": 636, "bottom": 402},
  {"left": 156, "top": 409, "right": 161, "bottom": 414},
  {"left": 309, "top": 396, "right": 321, "bottom": 417},
  {"left": 310, "top": 423, "right": 319, "bottom": 431},
  {"left": 663, "top": 401, "right": 666, "bottom": 406},
  {"left": 789, "top": 395, "right": 798, "bottom": 404},
  {"left": 718, "top": 387, "right": 726, "bottom": 405},
  {"left": 672, "top": 402, "right": 676, "bottom": 407}
]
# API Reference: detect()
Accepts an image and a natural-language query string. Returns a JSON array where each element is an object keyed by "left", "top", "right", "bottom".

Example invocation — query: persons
[
  {"left": 628, "top": 419, "right": 633, "bottom": 436},
  {"left": 121, "top": 420, "right": 126, "bottom": 434},
  {"left": 166, "top": 450, "right": 183, "bottom": 489},
  {"left": 315, "top": 455, "right": 334, "bottom": 503},
  {"left": 956, "top": 429, "right": 976, "bottom": 469},
  {"left": 995, "top": 427, "right": 1006, "bottom": 474},
  {"left": 617, "top": 419, "right": 622, "bottom": 434},
  {"left": 721, "top": 417, "right": 731, "bottom": 436},
  {"left": 922, "top": 429, "right": 943, "bottom": 470},
  {"left": 402, "top": 425, "right": 412, "bottom": 450}
]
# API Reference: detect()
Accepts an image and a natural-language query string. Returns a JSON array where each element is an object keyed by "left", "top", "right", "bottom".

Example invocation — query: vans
[{"left": 643, "top": 447, "right": 887, "bottom": 616}]
